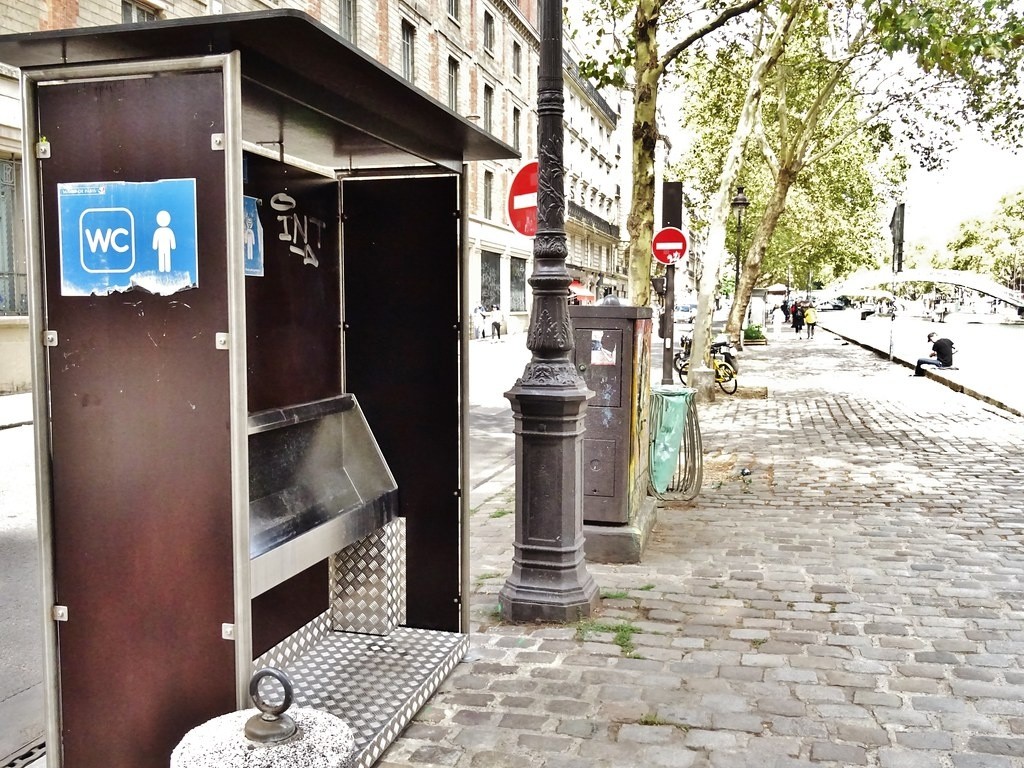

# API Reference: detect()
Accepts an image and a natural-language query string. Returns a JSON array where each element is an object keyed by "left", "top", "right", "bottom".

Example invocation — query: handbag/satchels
[{"left": 791, "top": 306, "right": 796, "bottom": 312}]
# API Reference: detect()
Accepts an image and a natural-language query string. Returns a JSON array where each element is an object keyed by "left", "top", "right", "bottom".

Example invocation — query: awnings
[{"left": 567, "top": 280, "right": 596, "bottom": 304}]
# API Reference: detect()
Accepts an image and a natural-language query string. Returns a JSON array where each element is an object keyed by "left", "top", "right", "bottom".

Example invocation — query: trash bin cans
[{"left": 649, "top": 385, "right": 703, "bottom": 501}]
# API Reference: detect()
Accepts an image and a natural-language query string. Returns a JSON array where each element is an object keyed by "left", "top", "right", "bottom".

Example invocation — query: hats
[{"left": 928, "top": 332, "right": 936, "bottom": 342}]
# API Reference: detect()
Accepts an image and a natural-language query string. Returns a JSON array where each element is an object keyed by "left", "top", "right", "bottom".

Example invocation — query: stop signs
[
  {"left": 652, "top": 227, "right": 689, "bottom": 266},
  {"left": 505, "top": 160, "right": 539, "bottom": 239}
]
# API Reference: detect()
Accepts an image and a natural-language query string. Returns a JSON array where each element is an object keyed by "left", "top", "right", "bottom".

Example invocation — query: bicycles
[{"left": 672, "top": 328, "right": 738, "bottom": 395}]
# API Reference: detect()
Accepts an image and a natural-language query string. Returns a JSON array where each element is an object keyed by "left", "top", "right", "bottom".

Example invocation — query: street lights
[{"left": 729, "top": 185, "right": 750, "bottom": 298}]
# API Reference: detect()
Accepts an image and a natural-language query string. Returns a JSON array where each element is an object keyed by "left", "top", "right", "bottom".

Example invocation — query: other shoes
[
  {"left": 842, "top": 342, "right": 849, "bottom": 345},
  {"left": 834, "top": 338, "right": 840, "bottom": 340}
]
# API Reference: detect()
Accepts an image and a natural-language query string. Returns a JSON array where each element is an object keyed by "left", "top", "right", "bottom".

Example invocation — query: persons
[
  {"left": 474, "top": 304, "right": 503, "bottom": 344},
  {"left": 781, "top": 300, "right": 817, "bottom": 341},
  {"left": 913, "top": 332, "right": 959, "bottom": 377}
]
